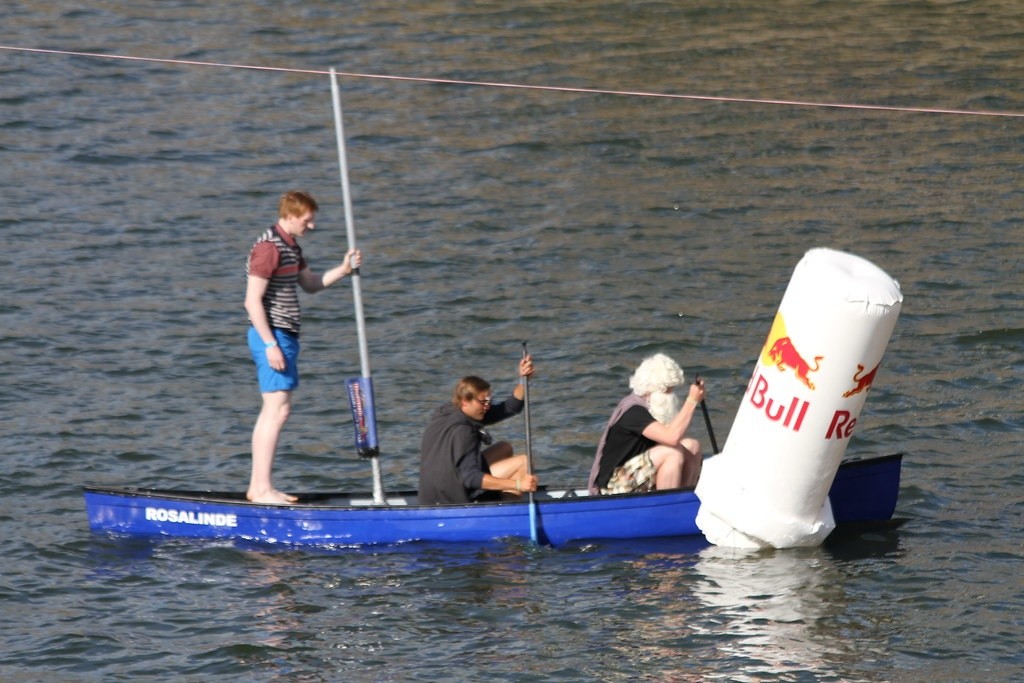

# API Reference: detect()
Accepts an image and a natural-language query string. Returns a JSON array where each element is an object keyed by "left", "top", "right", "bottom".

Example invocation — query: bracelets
[
  {"left": 686, "top": 398, "right": 696, "bottom": 405},
  {"left": 519, "top": 379, "right": 524, "bottom": 384},
  {"left": 265, "top": 341, "right": 277, "bottom": 348},
  {"left": 517, "top": 480, "right": 521, "bottom": 491}
]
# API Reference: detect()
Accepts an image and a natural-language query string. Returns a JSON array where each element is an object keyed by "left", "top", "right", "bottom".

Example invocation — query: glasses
[{"left": 472, "top": 399, "right": 493, "bottom": 407}]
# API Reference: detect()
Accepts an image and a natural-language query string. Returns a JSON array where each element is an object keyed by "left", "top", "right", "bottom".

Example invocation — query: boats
[{"left": 80, "top": 449, "right": 905, "bottom": 547}]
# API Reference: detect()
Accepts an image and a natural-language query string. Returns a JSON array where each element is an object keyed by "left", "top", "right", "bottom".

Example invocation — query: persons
[
  {"left": 418, "top": 354, "right": 538, "bottom": 504},
  {"left": 587, "top": 353, "right": 706, "bottom": 495},
  {"left": 243, "top": 192, "right": 361, "bottom": 504}
]
